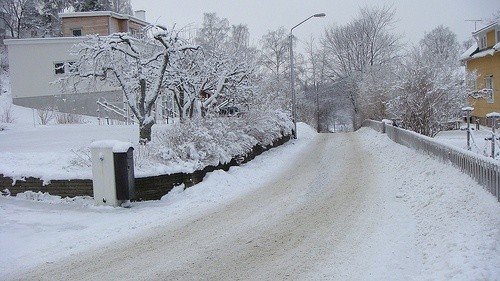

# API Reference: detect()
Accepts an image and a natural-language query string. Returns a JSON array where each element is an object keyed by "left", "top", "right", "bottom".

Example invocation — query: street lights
[{"left": 289, "top": 12, "right": 327, "bottom": 141}]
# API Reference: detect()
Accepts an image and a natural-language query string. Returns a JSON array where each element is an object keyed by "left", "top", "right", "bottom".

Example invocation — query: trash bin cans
[
  {"left": 90, "top": 138, "right": 124, "bottom": 206},
  {"left": 113, "top": 141, "right": 136, "bottom": 202}
]
